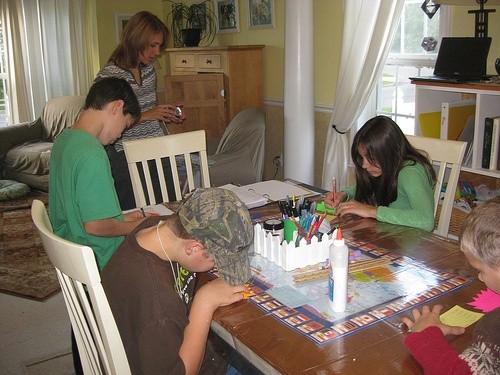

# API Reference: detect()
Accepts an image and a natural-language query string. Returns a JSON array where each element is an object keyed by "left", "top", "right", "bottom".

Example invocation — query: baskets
[{"left": 434, "top": 204, "right": 468, "bottom": 236}]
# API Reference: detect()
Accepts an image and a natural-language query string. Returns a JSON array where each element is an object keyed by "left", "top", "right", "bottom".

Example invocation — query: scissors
[{"left": 295, "top": 213, "right": 315, "bottom": 247}]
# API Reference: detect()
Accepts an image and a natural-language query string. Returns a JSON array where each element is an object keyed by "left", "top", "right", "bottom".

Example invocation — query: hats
[{"left": 176, "top": 187, "right": 255, "bottom": 286}]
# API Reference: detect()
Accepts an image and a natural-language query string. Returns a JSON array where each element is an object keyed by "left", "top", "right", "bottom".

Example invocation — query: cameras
[{"left": 166, "top": 103, "right": 182, "bottom": 118}]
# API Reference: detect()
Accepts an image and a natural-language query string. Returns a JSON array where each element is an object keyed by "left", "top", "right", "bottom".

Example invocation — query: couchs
[
  {"left": 163, "top": 107, "right": 266, "bottom": 193},
  {"left": 0, "top": 97, "right": 90, "bottom": 194}
]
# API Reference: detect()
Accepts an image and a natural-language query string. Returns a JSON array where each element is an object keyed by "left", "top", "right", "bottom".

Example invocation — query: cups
[{"left": 282, "top": 218, "right": 300, "bottom": 245}]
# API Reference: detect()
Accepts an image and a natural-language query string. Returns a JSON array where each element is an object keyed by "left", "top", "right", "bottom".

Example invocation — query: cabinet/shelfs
[
  {"left": 164, "top": 44, "right": 265, "bottom": 135},
  {"left": 409, "top": 77, "right": 500, "bottom": 240}
]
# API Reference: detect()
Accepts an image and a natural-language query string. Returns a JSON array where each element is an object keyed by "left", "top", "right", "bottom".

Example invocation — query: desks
[{"left": 122, "top": 178, "right": 500, "bottom": 375}]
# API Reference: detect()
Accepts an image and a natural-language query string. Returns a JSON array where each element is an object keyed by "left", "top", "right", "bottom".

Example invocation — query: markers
[
  {"left": 299, "top": 198, "right": 316, "bottom": 218},
  {"left": 278, "top": 195, "right": 299, "bottom": 221}
]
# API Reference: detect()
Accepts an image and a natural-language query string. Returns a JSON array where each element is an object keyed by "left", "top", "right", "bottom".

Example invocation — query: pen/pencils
[
  {"left": 307, "top": 217, "right": 316, "bottom": 237},
  {"left": 250, "top": 216, "right": 262, "bottom": 219},
  {"left": 291, "top": 216, "right": 310, "bottom": 244},
  {"left": 327, "top": 224, "right": 339, "bottom": 235},
  {"left": 318, "top": 233, "right": 321, "bottom": 242},
  {"left": 140, "top": 207, "right": 146, "bottom": 218},
  {"left": 310, "top": 211, "right": 326, "bottom": 238},
  {"left": 333, "top": 177, "right": 336, "bottom": 203}
]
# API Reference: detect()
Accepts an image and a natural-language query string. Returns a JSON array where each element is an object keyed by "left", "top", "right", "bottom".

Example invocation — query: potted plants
[{"left": 166, "top": 0, "right": 215, "bottom": 48}]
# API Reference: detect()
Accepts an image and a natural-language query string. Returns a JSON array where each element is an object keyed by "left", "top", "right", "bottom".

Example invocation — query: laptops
[{"left": 409, "top": 35, "right": 492, "bottom": 85}]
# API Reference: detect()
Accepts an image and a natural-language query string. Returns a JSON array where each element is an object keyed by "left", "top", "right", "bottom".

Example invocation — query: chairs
[
  {"left": 30, "top": 198, "right": 131, "bottom": 375},
  {"left": 405, "top": 135, "right": 468, "bottom": 239},
  {"left": 124, "top": 129, "right": 211, "bottom": 209}
]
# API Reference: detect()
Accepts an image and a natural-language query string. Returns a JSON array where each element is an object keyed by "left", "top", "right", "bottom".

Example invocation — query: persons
[
  {"left": 95, "top": 11, "right": 185, "bottom": 211},
  {"left": 325, "top": 116, "right": 435, "bottom": 232},
  {"left": 49, "top": 77, "right": 159, "bottom": 375},
  {"left": 100, "top": 187, "right": 252, "bottom": 374},
  {"left": 402, "top": 196, "right": 500, "bottom": 375}
]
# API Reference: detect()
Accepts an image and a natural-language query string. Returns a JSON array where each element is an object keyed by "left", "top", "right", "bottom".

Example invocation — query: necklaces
[{"left": 157, "top": 219, "right": 181, "bottom": 297}]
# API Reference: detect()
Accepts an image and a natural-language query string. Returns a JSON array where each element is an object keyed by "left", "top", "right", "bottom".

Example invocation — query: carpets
[{"left": 0, "top": 196, "right": 61, "bottom": 301}]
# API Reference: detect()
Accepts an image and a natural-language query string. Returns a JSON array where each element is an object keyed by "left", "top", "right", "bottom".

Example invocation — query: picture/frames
[
  {"left": 192, "top": 1, "right": 211, "bottom": 32},
  {"left": 214, "top": 0, "right": 240, "bottom": 34},
  {"left": 172, "top": 3, "right": 183, "bottom": 27},
  {"left": 245, "top": 0, "right": 275, "bottom": 30},
  {"left": 114, "top": 12, "right": 137, "bottom": 46}
]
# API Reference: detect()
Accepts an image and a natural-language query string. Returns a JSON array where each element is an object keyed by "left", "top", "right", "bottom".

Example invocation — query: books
[
  {"left": 482, "top": 117, "right": 500, "bottom": 173},
  {"left": 420, "top": 100, "right": 476, "bottom": 168}
]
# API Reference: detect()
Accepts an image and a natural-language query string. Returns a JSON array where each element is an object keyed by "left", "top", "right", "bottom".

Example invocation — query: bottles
[
  {"left": 328, "top": 228, "right": 349, "bottom": 313},
  {"left": 262, "top": 219, "right": 284, "bottom": 245}
]
